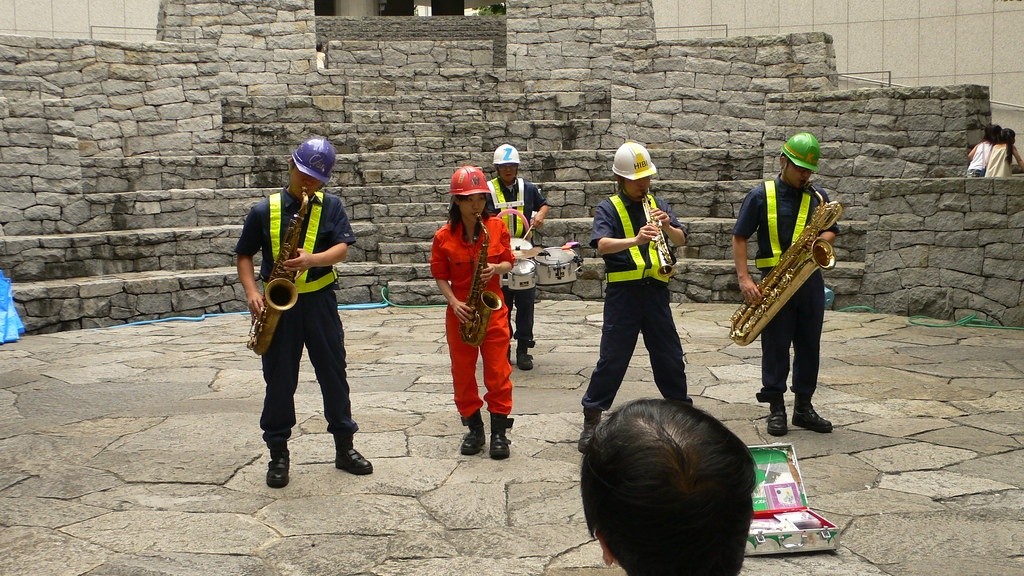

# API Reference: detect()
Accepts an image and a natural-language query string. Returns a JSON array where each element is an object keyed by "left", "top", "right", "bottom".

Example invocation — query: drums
[
  {"left": 507, "top": 259, "right": 537, "bottom": 291},
  {"left": 509, "top": 237, "right": 533, "bottom": 251},
  {"left": 534, "top": 246, "right": 579, "bottom": 287}
]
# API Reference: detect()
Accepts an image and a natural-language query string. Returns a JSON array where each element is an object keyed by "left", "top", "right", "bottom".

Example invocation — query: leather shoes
[
  {"left": 336, "top": 448, "right": 373, "bottom": 475},
  {"left": 792, "top": 408, "right": 833, "bottom": 432},
  {"left": 767, "top": 411, "right": 788, "bottom": 436},
  {"left": 266, "top": 455, "right": 290, "bottom": 488}
]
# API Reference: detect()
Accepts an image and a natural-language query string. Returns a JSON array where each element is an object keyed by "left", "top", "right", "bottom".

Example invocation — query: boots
[
  {"left": 507, "top": 344, "right": 511, "bottom": 364},
  {"left": 460, "top": 409, "right": 486, "bottom": 455},
  {"left": 489, "top": 412, "right": 514, "bottom": 458},
  {"left": 578, "top": 408, "right": 601, "bottom": 453},
  {"left": 516, "top": 340, "right": 535, "bottom": 371}
]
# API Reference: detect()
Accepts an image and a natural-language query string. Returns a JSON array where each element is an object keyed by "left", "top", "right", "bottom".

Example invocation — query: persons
[
  {"left": 431, "top": 166, "right": 516, "bottom": 459},
  {"left": 317, "top": 43, "right": 325, "bottom": 69},
  {"left": 233, "top": 138, "right": 373, "bottom": 488},
  {"left": 578, "top": 142, "right": 692, "bottom": 452},
  {"left": 580, "top": 399, "right": 756, "bottom": 576},
  {"left": 967, "top": 125, "right": 1024, "bottom": 177},
  {"left": 486, "top": 144, "right": 549, "bottom": 369},
  {"left": 732, "top": 133, "right": 841, "bottom": 435}
]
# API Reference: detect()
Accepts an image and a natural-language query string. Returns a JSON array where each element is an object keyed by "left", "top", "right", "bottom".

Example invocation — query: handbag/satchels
[{"left": 0, "top": 270, "right": 25, "bottom": 344}]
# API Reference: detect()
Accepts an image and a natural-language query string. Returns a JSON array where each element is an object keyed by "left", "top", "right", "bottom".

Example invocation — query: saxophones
[
  {"left": 245, "top": 184, "right": 310, "bottom": 356},
  {"left": 458, "top": 213, "right": 503, "bottom": 347},
  {"left": 727, "top": 179, "right": 844, "bottom": 347},
  {"left": 641, "top": 189, "right": 675, "bottom": 278}
]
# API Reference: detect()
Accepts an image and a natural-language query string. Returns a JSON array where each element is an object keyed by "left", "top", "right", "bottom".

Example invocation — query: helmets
[
  {"left": 782, "top": 133, "right": 820, "bottom": 172},
  {"left": 292, "top": 137, "right": 335, "bottom": 184},
  {"left": 449, "top": 166, "right": 492, "bottom": 197},
  {"left": 493, "top": 144, "right": 520, "bottom": 165},
  {"left": 612, "top": 141, "right": 657, "bottom": 181}
]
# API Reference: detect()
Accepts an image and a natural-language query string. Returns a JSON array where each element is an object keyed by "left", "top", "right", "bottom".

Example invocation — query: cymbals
[{"left": 513, "top": 248, "right": 538, "bottom": 259}]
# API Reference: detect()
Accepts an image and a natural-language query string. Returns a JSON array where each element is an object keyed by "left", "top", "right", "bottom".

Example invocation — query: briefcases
[{"left": 742, "top": 441, "right": 839, "bottom": 557}]
